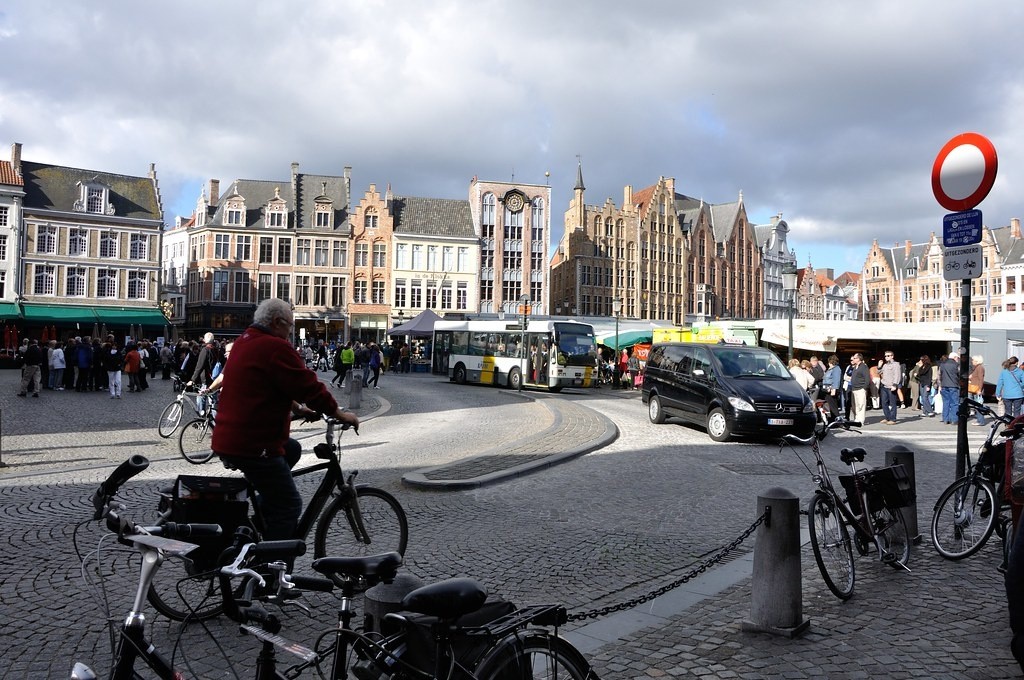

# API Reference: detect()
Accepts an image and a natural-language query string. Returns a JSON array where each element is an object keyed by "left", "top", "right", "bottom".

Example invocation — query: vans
[{"left": 641, "top": 342, "right": 817, "bottom": 443}]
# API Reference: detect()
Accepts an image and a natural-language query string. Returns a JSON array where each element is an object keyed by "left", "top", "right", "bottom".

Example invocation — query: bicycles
[
  {"left": 156, "top": 374, "right": 219, "bottom": 465},
  {"left": 806, "top": 390, "right": 828, "bottom": 436},
  {"left": 778, "top": 415, "right": 913, "bottom": 600},
  {"left": 140, "top": 411, "right": 409, "bottom": 623},
  {"left": 73, "top": 456, "right": 602, "bottom": 680},
  {"left": 931, "top": 396, "right": 1024, "bottom": 561}
]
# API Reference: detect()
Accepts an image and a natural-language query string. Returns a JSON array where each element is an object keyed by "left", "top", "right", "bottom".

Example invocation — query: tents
[{"left": 386, "top": 309, "right": 442, "bottom": 374}]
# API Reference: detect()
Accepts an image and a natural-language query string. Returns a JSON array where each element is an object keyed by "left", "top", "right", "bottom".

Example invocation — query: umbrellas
[
  {"left": 12, "top": 325, "right": 18, "bottom": 359},
  {"left": 51, "top": 325, "right": 56, "bottom": 340},
  {"left": 42, "top": 326, "right": 48, "bottom": 343},
  {"left": 5, "top": 326, "right": 10, "bottom": 355}
]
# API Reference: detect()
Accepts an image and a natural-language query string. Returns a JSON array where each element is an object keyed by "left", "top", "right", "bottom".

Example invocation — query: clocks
[{"left": 505, "top": 193, "right": 523, "bottom": 211}]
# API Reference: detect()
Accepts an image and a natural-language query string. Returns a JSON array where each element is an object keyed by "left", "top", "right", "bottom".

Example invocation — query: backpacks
[{"left": 210, "top": 348, "right": 223, "bottom": 380}]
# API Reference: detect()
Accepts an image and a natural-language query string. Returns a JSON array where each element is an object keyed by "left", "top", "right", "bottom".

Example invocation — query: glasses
[{"left": 885, "top": 355, "right": 893, "bottom": 357}]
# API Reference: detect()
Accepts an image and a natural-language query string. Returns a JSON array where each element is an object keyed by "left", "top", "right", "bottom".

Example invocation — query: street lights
[
  {"left": 324, "top": 315, "right": 330, "bottom": 344},
  {"left": 782, "top": 264, "right": 799, "bottom": 361},
  {"left": 397, "top": 310, "right": 404, "bottom": 342},
  {"left": 612, "top": 297, "right": 620, "bottom": 390}
]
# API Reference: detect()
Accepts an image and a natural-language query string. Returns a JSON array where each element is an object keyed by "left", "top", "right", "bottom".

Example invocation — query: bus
[{"left": 431, "top": 320, "right": 599, "bottom": 392}]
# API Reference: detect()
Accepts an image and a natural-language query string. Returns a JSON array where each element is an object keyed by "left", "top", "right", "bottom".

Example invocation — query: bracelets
[{"left": 209, "top": 388, "right": 212, "bottom": 392}]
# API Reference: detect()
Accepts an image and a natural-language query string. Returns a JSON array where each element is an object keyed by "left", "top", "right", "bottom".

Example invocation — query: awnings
[
  {"left": 94, "top": 309, "right": 168, "bottom": 325},
  {"left": 0, "top": 304, "right": 23, "bottom": 319},
  {"left": 23, "top": 306, "right": 99, "bottom": 322},
  {"left": 603, "top": 331, "right": 653, "bottom": 350}
]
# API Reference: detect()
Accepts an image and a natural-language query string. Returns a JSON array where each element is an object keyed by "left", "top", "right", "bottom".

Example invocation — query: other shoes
[
  {"left": 944, "top": 420, "right": 948, "bottom": 425},
  {"left": 18, "top": 376, "right": 171, "bottom": 398},
  {"left": 880, "top": 419, "right": 895, "bottom": 425},
  {"left": 307, "top": 366, "right": 407, "bottom": 389},
  {"left": 920, "top": 412, "right": 933, "bottom": 417},
  {"left": 971, "top": 422, "right": 985, "bottom": 426},
  {"left": 253, "top": 583, "right": 303, "bottom": 599},
  {"left": 951, "top": 421, "right": 958, "bottom": 424},
  {"left": 900, "top": 404, "right": 906, "bottom": 410}
]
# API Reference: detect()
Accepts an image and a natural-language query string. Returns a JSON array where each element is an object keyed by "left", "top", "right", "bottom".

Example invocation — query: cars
[{"left": 0, "top": 349, "right": 18, "bottom": 358}]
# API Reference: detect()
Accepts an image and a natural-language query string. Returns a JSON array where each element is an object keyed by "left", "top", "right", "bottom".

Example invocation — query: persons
[
  {"left": 766, "top": 356, "right": 826, "bottom": 404},
  {"left": 822, "top": 353, "right": 870, "bottom": 427},
  {"left": 909, "top": 352, "right": 985, "bottom": 426},
  {"left": 995, "top": 356, "right": 1024, "bottom": 423},
  {"left": 869, "top": 351, "right": 906, "bottom": 424},
  {"left": 186, "top": 332, "right": 226, "bottom": 427},
  {"left": 297, "top": 338, "right": 409, "bottom": 390},
  {"left": 199, "top": 342, "right": 233, "bottom": 394},
  {"left": 618, "top": 349, "right": 639, "bottom": 391},
  {"left": 211, "top": 299, "right": 359, "bottom": 600},
  {"left": 17, "top": 334, "right": 124, "bottom": 398},
  {"left": 596, "top": 348, "right": 605, "bottom": 388},
  {"left": 602, "top": 348, "right": 611, "bottom": 363},
  {"left": 124, "top": 337, "right": 204, "bottom": 393}
]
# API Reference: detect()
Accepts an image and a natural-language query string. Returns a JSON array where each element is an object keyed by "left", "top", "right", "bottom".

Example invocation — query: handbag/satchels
[
  {"left": 968, "top": 383, "right": 980, "bottom": 393},
  {"left": 998, "top": 400, "right": 1005, "bottom": 416},
  {"left": 622, "top": 371, "right": 629, "bottom": 382},
  {"left": 931, "top": 387, "right": 944, "bottom": 414},
  {"left": 124, "top": 362, "right": 131, "bottom": 372},
  {"left": 333, "top": 363, "right": 337, "bottom": 372}
]
